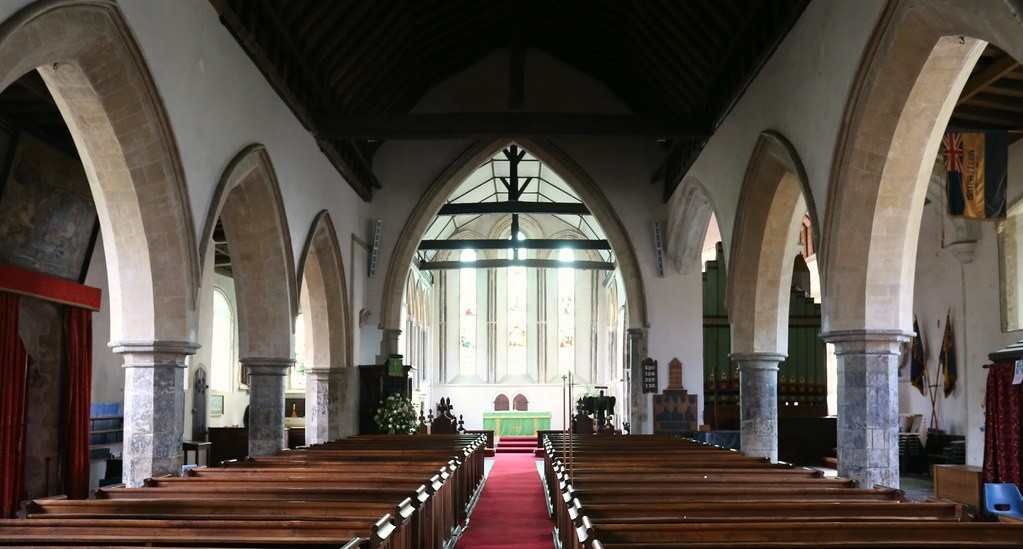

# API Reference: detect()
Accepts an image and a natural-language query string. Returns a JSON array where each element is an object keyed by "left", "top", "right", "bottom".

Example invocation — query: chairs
[{"left": 984, "top": 482, "right": 1023, "bottom": 518}]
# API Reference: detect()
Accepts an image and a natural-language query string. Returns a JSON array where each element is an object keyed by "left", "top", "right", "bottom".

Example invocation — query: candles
[{"left": 293, "top": 404, "right": 295, "bottom": 411}]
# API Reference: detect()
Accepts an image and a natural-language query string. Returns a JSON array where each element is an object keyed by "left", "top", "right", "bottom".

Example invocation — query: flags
[
  {"left": 939, "top": 314, "right": 958, "bottom": 398},
  {"left": 910, "top": 320, "right": 926, "bottom": 396}
]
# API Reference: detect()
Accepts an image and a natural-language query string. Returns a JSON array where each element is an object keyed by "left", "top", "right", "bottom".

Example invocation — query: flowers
[{"left": 374, "top": 393, "right": 419, "bottom": 435}]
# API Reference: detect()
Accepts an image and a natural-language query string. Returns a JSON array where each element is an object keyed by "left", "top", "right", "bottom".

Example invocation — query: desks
[
  {"left": 184, "top": 440, "right": 212, "bottom": 468},
  {"left": 483, "top": 410, "right": 552, "bottom": 437},
  {"left": 932, "top": 464, "right": 983, "bottom": 511}
]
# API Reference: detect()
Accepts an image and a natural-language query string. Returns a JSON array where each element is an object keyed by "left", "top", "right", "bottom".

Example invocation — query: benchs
[
  {"left": 0, "top": 434, "right": 488, "bottom": 549},
  {"left": 542, "top": 433, "right": 1023, "bottom": 549}
]
